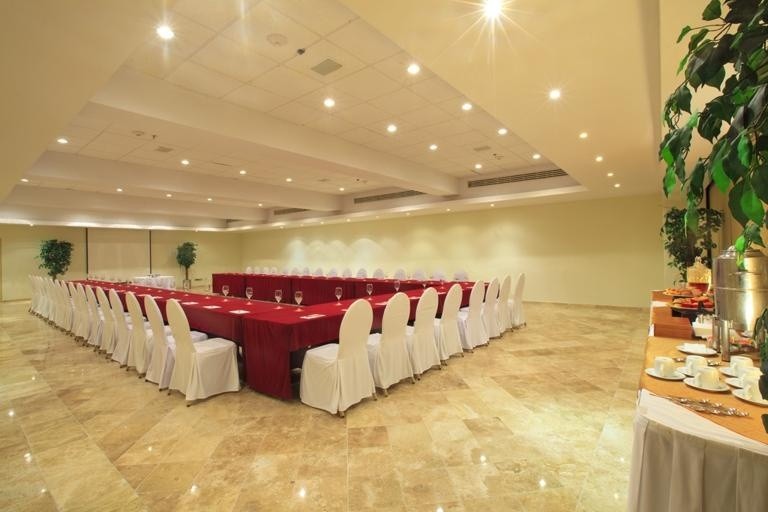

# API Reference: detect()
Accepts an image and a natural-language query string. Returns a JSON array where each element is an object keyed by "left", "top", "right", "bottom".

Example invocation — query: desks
[
  {"left": 213, "top": 273, "right": 457, "bottom": 306},
  {"left": 65, "top": 279, "right": 307, "bottom": 402},
  {"left": 242, "top": 281, "right": 501, "bottom": 401}
]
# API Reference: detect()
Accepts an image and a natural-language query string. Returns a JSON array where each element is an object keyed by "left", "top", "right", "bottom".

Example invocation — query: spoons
[
  {"left": 650, "top": 394, "right": 723, "bottom": 414},
  {"left": 721, "top": 408, "right": 748, "bottom": 417}
]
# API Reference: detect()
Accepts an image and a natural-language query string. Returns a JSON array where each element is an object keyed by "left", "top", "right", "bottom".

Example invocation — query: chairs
[
  {"left": 498, "top": 274, "right": 513, "bottom": 337},
  {"left": 77, "top": 283, "right": 92, "bottom": 345},
  {"left": 96, "top": 287, "right": 145, "bottom": 360},
  {"left": 300, "top": 299, "right": 378, "bottom": 418},
  {"left": 461, "top": 277, "right": 499, "bottom": 342},
  {"left": 108, "top": 288, "right": 152, "bottom": 367},
  {"left": 126, "top": 291, "right": 171, "bottom": 378},
  {"left": 367, "top": 292, "right": 416, "bottom": 397},
  {"left": 143, "top": 296, "right": 207, "bottom": 392},
  {"left": 435, "top": 284, "right": 464, "bottom": 366},
  {"left": 458, "top": 280, "right": 487, "bottom": 353},
  {"left": 245, "top": 268, "right": 468, "bottom": 279},
  {"left": 85, "top": 285, "right": 104, "bottom": 352},
  {"left": 512, "top": 271, "right": 525, "bottom": 329},
  {"left": 167, "top": 299, "right": 241, "bottom": 407},
  {"left": 408, "top": 288, "right": 442, "bottom": 381},
  {"left": 28, "top": 274, "right": 72, "bottom": 335},
  {"left": 69, "top": 282, "right": 79, "bottom": 340}
]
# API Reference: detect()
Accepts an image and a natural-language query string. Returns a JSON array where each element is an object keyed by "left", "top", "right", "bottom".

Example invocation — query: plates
[
  {"left": 721, "top": 366, "right": 764, "bottom": 379},
  {"left": 725, "top": 377, "right": 759, "bottom": 389},
  {"left": 732, "top": 389, "right": 767, "bottom": 406},
  {"left": 645, "top": 367, "right": 685, "bottom": 381},
  {"left": 678, "top": 367, "right": 696, "bottom": 377},
  {"left": 684, "top": 377, "right": 730, "bottom": 391},
  {"left": 678, "top": 346, "right": 716, "bottom": 357}
]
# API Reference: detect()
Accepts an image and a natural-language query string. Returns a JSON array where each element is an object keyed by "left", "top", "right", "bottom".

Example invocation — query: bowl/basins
[{"left": 653, "top": 356, "right": 674, "bottom": 376}]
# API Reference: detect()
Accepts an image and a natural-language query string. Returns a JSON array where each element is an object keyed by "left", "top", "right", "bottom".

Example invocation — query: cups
[
  {"left": 745, "top": 382, "right": 763, "bottom": 400},
  {"left": 246, "top": 287, "right": 253, "bottom": 306},
  {"left": 274, "top": 290, "right": 283, "bottom": 310},
  {"left": 455, "top": 276, "right": 458, "bottom": 284},
  {"left": 422, "top": 278, "right": 426, "bottom": 290},
  {"left": 125, "top": 279, "right": 131, "bottom": 288},
  {"left": 100, "top": 273, "right": 105, "bottom": 285},
  {"left": 110, "top": 274, "right": 115, "bottom": 285},
  {"left": 362, "top": 273, "right": 366, "bottom": 282},
  {"left": 146, "top": 275, "right": 154, "bottom": 290},
  {"left": 222, "top": 284, "right": 230, "bottom": 302},
  {"left": 694, "top": 367, "right": 720, "bottom": 388},
  {"left": 406, "top": 274, "right": 410, "bottom": 284},
  {"left": 133, "top": 278, "right": 139, "bottom": 290},
  {"left": 117, "top": 277, "right": 122, "bottom": 287},
  {"left": 384, "top": 273, "right": 388, "bottom": 282},
  {"left": 156, "top": 277, "right": 163, "bottom": 293},
  {"left": 367, "top": 284, "right": 373, "bottom": 301},
  {"left": 169, "top": 278, "right": 176, "bottom": 294},
  {"left": 335, "top": 287, "right": 344, "bottom": 307},
  {"left": 294, "top": 291, "right": 303, "bottom": 311},
  {"left": 730, "top": 356, "right": 753, "bottom": 374},
  {"left": 394, "top": 281, "right": 400, "bottom": 293},
  {"left": 203, "top": 284, "right": 212, "bottom": 300},
  {"left": 739, "top": 367, "right": 761, "bottom": 386},
  {"left": 440, "top": 279, "right": 444, "bottom": 288},
  {"left": 685, "top": 356, "right": 706, "bottom": 371},
  {"left": 183, "top": 280, "right": 190, "bottom": 296}
]
